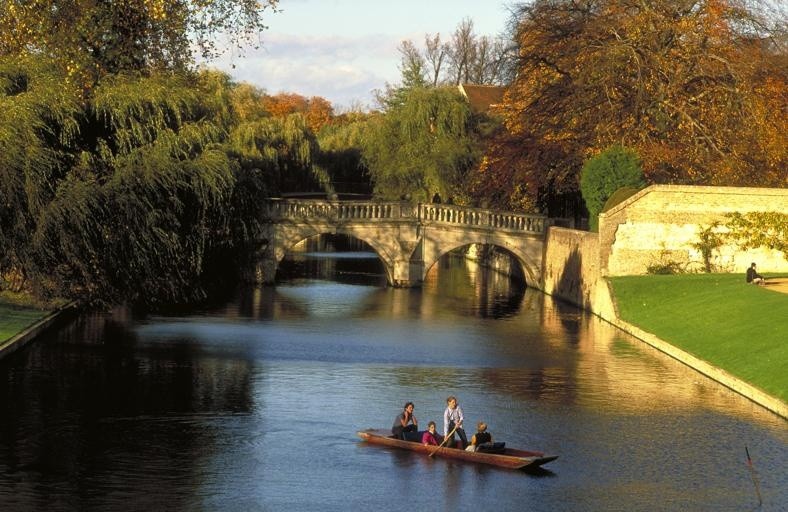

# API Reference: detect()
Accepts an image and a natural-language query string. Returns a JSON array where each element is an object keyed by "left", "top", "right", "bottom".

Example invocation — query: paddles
[{"left": 428, "top": 425, "right": 457, "bottom": 457}]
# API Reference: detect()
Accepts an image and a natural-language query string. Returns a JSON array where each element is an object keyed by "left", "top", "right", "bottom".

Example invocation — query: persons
[
  {"left": 422, "top": 421, "right": 443, "bottom": 447},
  {"left": 464, "top": 422, "right": 494, "bottom": 452},
  {"left": 432, "top": 192, "right": 454, "bottom": 215},
  {"left": 746, "top": 263, "right": 765, "bottom": 286},
  {"left": 443, "top": 395, "right": 468, "bottom": 449},
  {"left": 391, "top": 402, "right": 417, "bottom": 440}
]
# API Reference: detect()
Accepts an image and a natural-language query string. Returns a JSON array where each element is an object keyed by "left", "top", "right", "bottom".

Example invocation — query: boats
[{"left": 355, "top": 428, "right": 559, "bottom": 470}]
